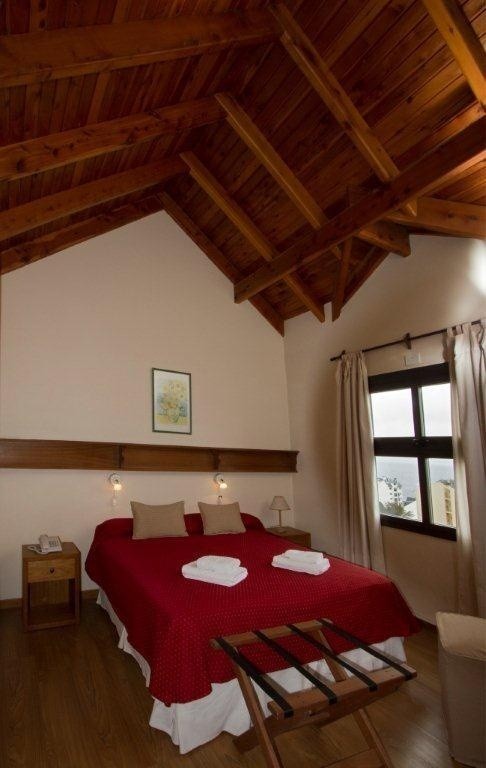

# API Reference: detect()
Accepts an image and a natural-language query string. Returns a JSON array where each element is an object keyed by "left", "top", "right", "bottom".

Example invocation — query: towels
[
  {"left": 181, "top": 555, "right": 248, "bottom": 587},
  {"left": 271, "top": 549, "right": 330, "bottom": 576}
]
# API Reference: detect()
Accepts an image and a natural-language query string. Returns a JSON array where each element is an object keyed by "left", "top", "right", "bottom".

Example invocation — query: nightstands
[
  {"left": 265, "top": 526, "right": 311, "bottom": 549},
  {"left": 22, "top": 542, "right": 81, "bottom": 633}
]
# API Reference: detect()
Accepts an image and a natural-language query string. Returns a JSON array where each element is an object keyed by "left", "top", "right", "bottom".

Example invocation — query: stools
[
  {"left": 435, "top": 612, "right": 486, "bottom": 767},
  {"left": 210, "top": 617, "right": 417, "bottom": 768}
]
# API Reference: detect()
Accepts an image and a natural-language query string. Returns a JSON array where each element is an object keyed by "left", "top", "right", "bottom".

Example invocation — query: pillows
[
  {"left": 197, "top": 502, "right": 246, "bottom": 535},
  {"left": 129, "top": 500, "right": 190, "bottom": 540}
]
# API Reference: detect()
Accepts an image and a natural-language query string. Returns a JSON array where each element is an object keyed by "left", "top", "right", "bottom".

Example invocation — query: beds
[{"left": 85, "top": 513, "right": 422, "bottom": 755}]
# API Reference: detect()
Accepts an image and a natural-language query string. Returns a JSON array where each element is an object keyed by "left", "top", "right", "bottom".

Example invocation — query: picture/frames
[{"left": 152, "top": 368, "right": 191, "bottom": 434}]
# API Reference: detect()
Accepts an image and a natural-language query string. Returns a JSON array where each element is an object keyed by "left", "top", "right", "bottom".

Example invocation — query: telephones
[{"left": 39, "top": 534, "right": 62, "bottom": 552}]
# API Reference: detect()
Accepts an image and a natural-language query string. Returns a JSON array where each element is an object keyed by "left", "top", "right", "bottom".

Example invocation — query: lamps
[
  {"left": 269, "top": 496, "right": 291, "bottom": 532},
  {"left": 110, "top": 474, "right": 122, "bottom": 507},
  {"left": 216, "top": 474, "right": 228, "bottom": 503}
]
